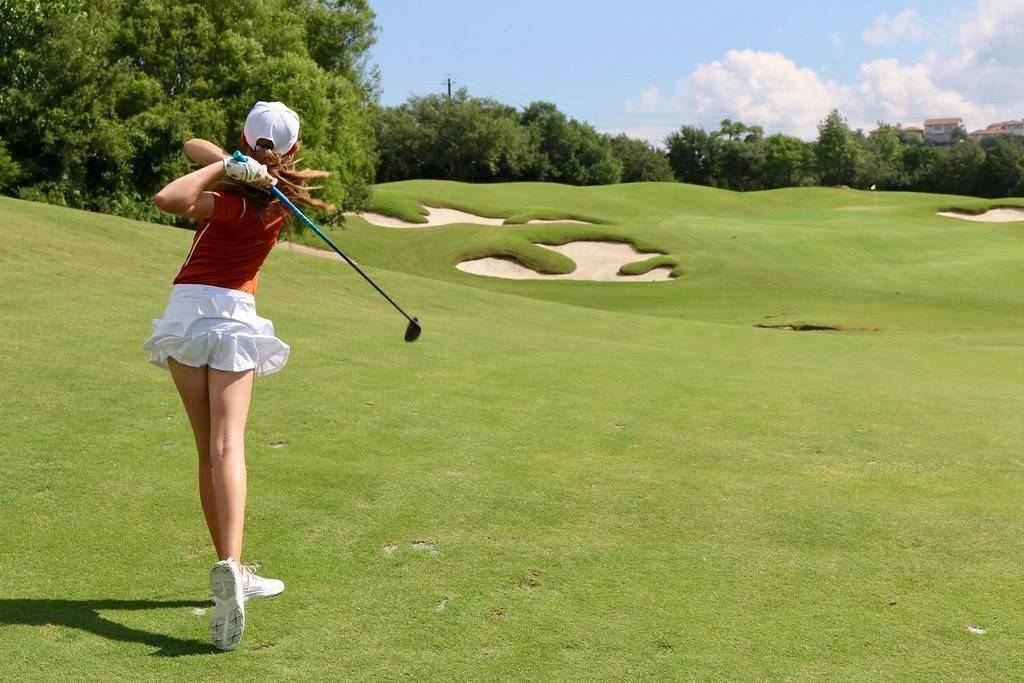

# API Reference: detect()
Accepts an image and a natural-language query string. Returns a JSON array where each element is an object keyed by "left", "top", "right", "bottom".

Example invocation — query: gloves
[{"left": 222, "top": 156, "right": 267, "bottom": 182}]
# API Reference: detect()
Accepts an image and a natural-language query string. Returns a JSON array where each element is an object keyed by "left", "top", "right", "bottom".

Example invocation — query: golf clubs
[{"left": 232, "top": 150, "right": 423, "bottom": 343}]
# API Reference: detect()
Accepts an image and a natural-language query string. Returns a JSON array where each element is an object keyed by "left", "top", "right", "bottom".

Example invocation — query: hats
[{"left": 243, "top": 101, "right": 300, "bottom": 158}]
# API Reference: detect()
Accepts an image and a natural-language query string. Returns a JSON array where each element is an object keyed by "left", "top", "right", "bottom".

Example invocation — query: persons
[{"left": 153, "top": 101, "right": 331, "bottom": 650}]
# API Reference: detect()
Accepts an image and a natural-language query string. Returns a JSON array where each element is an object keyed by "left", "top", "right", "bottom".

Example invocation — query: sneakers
[
  {"left": 241, "top": 562, "right": 285, "bottom": 602},
  {"left": 210, "top": 556, "right": 244, "bottom": 650}
]
